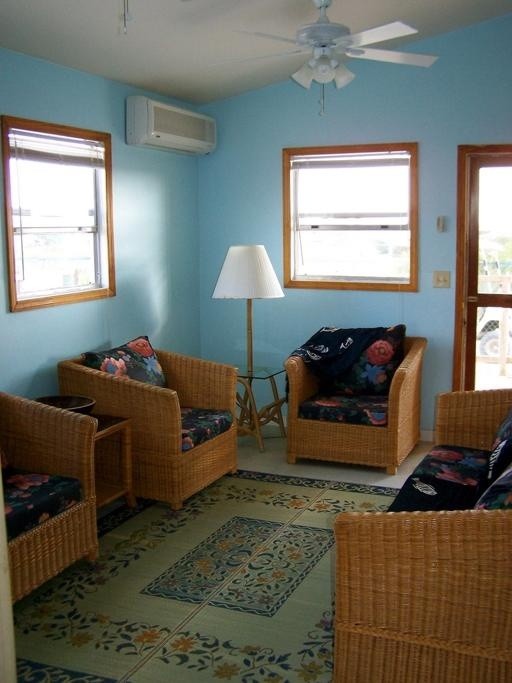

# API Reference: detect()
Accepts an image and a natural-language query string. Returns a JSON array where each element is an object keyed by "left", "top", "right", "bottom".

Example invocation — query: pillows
[
  {"left": 319, "top": 324, "right": 407, "bottom": 398},
  {"left": 79, "top": 335, "right": 169, "bottom": 389}
]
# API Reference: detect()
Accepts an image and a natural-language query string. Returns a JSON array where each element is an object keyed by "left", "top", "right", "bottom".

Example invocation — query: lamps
[
  {"left": 289, "top": 44, "right": 356, "bottom": 89},
  {"left": 209, "top": 242, "right": 286, "bottom": 370}
]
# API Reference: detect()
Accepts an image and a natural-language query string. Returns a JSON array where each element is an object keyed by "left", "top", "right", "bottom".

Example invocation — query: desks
[{"left": 82, "top": 414, "right": 141, "bottom": 519}]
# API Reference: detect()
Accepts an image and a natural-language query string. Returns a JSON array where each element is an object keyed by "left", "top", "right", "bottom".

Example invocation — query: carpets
[{"left": 15, "top": 469, "right": 401, "bottom": 682}]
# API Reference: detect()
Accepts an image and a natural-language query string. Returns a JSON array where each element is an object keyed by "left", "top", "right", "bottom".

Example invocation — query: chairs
[
  {"left": 57, "top": 345, "right": 241, "bottom": 510},
  {"left": 1, "top": 392, "right": 100, "bottom": 602},
  {"left": 284, "top": 322, "right": 428, "bottom": 475}
]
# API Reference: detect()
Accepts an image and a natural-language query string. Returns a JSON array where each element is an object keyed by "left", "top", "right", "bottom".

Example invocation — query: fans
[{"left": 208, "top": 0, "right": 439, "bottom": 69}]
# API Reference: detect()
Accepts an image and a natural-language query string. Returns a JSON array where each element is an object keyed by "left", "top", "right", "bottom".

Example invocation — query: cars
[{"left": 476, "top": 306, "right": 512, "bottom": 356}]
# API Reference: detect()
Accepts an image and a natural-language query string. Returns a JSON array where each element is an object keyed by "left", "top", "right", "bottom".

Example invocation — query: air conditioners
[{"left": 125, "top": 91, "right": 218, "bottom": 156}]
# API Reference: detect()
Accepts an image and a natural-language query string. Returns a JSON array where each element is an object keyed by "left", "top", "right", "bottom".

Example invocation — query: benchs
[{"left": 331, "top": 386, "right": 511, "bottom": 680}]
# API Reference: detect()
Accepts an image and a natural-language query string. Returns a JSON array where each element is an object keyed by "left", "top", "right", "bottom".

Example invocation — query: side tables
[{"left": 235, "top": 367, "right": 288, "bottom": 451}]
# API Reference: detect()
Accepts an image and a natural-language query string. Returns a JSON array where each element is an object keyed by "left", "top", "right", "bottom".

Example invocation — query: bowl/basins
[{"left": 34, "top": 394, "right": 96, "bottom": 417}]
